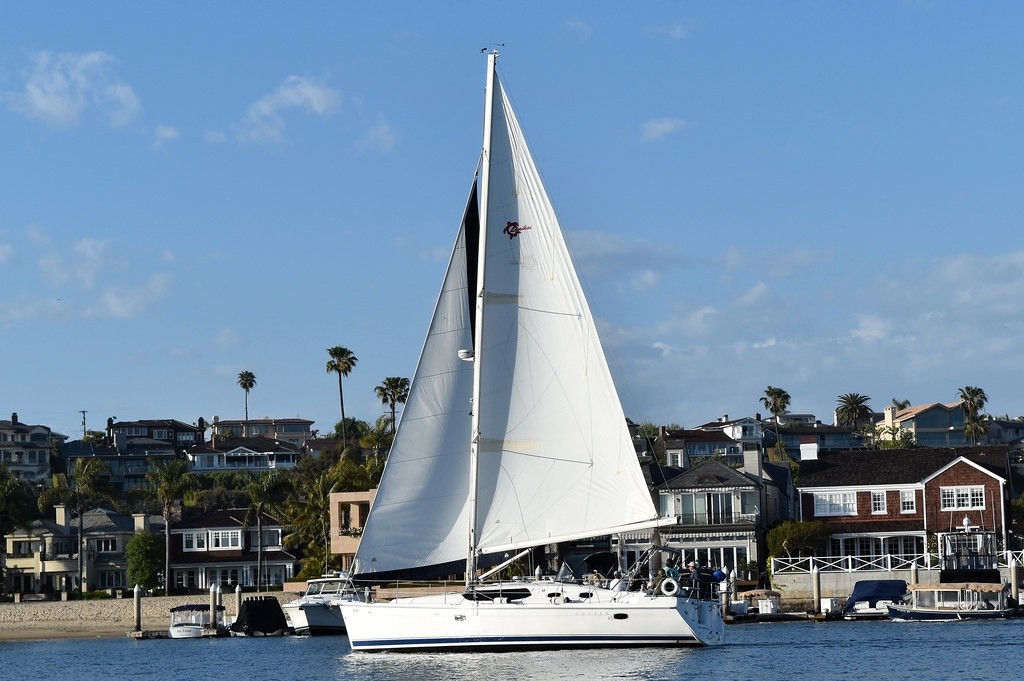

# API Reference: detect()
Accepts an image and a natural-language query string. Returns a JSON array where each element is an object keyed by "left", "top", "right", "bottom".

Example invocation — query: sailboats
[{"left": 326, "top": 48, "right": 735, "bottom": 655}]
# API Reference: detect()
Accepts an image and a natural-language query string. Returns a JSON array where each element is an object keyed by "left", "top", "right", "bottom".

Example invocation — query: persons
[
  {"left": 955, "top": 542, "right": 991, "bottom": 569},
  {"left": 610, "top": 571, "right": 626, "bottom": 591},
  {"left": 1006, "top": 594, "right": 1014, "bottom": 608},
  {"left": 337, "top": 525, "right": 364, "bottom": 538},
  {"left": 663, "top": 558, "right": 679, "bottom": 581},
  {"left": 594, "top": 574, "right": 602, "bottom": 588},
  {"left": 984, "top": 599, "right": 994, "bottom": 610},
  {"left": 652, "top": 568, "right": 669, "bottom": 595},
  {"left": 687, "top": 562, "right": 705, "bottom": 598}
]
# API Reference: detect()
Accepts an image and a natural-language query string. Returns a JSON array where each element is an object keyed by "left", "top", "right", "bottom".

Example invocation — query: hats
[{"left": 687, "top": 562, "right": 695, "bottom": 566}]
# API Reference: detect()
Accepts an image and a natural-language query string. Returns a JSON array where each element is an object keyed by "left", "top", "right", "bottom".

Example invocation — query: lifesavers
[{"left": 661, "top": 579, "right": 678, "bottom": 597}]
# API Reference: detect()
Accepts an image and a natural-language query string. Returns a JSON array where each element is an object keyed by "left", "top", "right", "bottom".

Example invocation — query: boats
[
  {"left": 887, "top": 583, "right": 1016, "bottom": 621},
  {"left": 841, "top": 578, "right": 906, "bottom": 620},
  {"left": 281, "top": 572, "right": 373, "bottom": 637},
  {"left": 230, "top": 597, "right": 293, "bottom": 637},
  {"left": 169, "top": 604, "right": 226, "bottom": 638}
]
